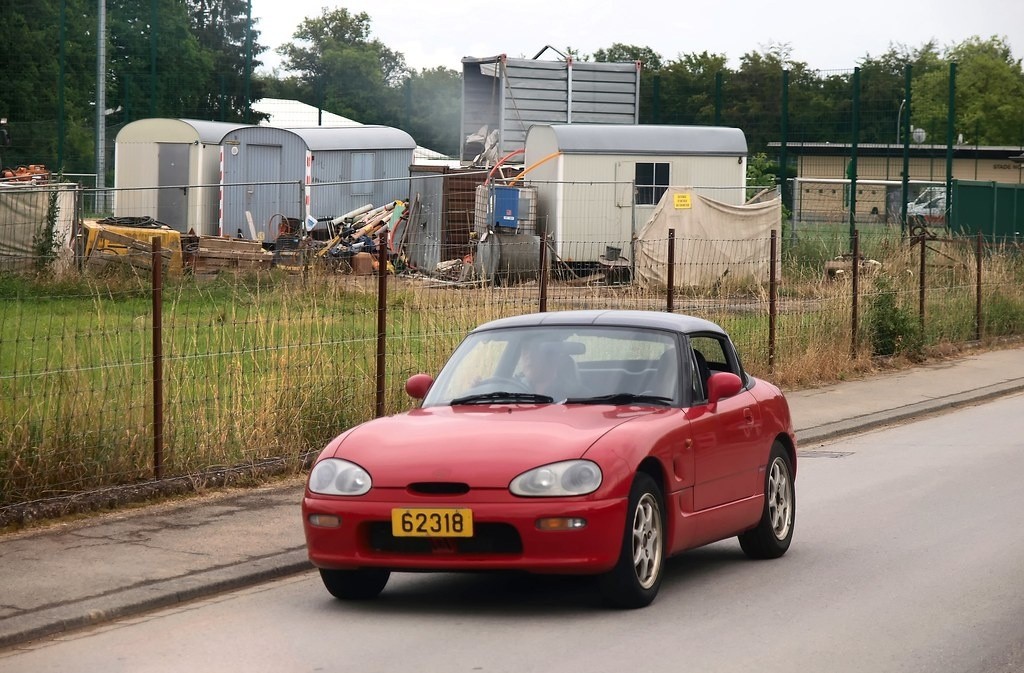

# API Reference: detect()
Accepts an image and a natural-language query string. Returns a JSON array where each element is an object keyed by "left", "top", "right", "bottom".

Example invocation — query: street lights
[{"left": 897, "top": 99, "right": 906, "bottom": 144}]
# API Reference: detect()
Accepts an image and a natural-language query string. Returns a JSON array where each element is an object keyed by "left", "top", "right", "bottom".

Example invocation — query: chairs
[{"left": 656, "top": 348, "right": 710, "bottom": 402}]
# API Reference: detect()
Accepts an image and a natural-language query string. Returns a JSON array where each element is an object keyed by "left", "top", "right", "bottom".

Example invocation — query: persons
[{"left": 467, "top": 331, "right": 596, "bottom": 402}]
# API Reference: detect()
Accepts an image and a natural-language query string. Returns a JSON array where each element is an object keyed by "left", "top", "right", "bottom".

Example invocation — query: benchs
[{"left": 556, "top": 352, "right": 659, "bottom": 394}]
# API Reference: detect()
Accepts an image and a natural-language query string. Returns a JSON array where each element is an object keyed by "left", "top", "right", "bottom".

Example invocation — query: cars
[
  {"left": 303, "top": 310, "right": 800, "bottom": 610},
  {"left": 906, "top": 186, "right": 946, "bottom": 211},
  {"left": 907, "top": 197, "right": 946, "bottom": 228}
]
